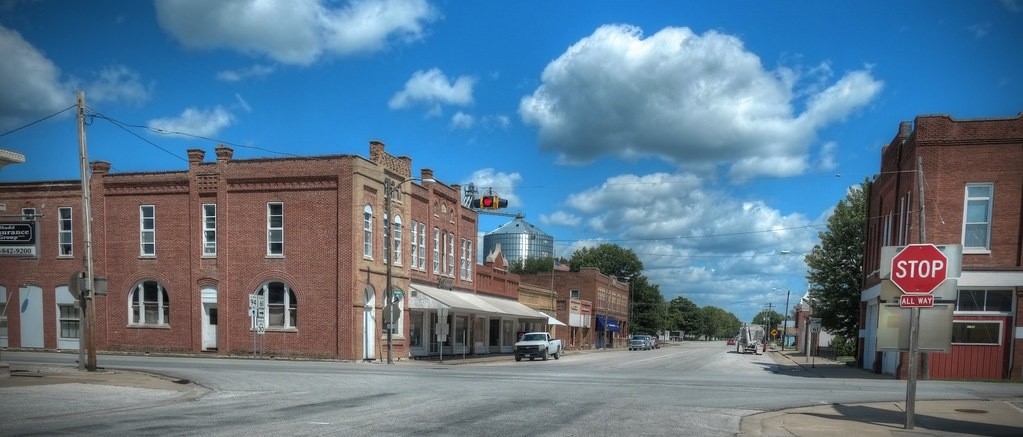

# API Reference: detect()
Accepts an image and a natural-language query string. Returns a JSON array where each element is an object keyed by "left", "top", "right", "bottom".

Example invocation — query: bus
[{"left": 669, "top": 331, "right": 683, "bottom": 341}]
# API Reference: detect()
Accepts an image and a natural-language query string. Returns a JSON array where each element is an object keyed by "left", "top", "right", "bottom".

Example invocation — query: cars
[
  {"left": 650, "top": 336, "right": 660, "bottom": 349},
  {"left": 629, "top": 335, "right": 652, "bottom": 351},
  {"left": 727, "top": 339, "right": 735, "bottom": 345}
]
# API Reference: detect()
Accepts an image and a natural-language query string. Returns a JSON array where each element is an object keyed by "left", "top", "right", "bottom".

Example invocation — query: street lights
[
  {"left": 781, "top": 251, "right": 815, "bottom": 362},
  {"left": 385, "top": 177, "right": 436, "bottom": 364},
  {"left": 772, "top": 287, "right": 790, "bottom": 350}
]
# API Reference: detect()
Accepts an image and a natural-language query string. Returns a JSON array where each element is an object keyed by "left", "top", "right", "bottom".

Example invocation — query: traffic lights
[{"left": 472, "top": 195, "right": 508, "bottom": 209}]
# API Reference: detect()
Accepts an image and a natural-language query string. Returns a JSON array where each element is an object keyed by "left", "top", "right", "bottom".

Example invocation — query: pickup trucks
[{"left": 514, "top": 332, "right": 562, "bottom": 361}]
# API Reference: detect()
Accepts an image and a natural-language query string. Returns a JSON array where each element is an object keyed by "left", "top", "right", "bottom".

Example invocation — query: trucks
[{"left": 736, "top": 323, "right": 767, "bottom": 354}]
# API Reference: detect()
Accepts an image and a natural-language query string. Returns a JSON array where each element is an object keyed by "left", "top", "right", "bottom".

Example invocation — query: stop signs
[{"left": 891, "top": 243, "right": 948, "bottom": 294}]
